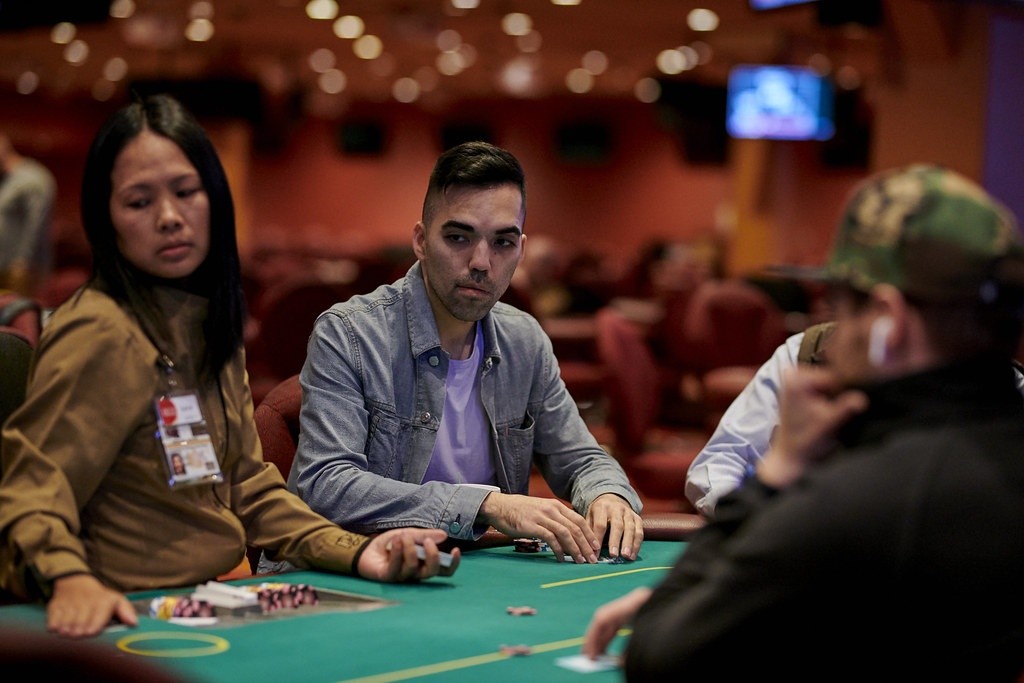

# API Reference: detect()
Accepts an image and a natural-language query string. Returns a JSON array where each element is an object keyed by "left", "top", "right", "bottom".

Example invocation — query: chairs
[{"left": 14, "top": 257, "right": 788, "bottom": 541}]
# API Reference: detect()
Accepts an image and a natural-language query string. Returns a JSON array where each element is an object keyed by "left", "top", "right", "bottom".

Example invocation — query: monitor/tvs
[{"left": 724, "top": 63, "right": 839, "bottom": 140}]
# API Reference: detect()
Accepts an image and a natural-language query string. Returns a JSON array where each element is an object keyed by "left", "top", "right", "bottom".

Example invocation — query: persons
[
  {"left": 0, "top": 94, "right": 459, "bottom": 639},
  {"left": 585, "top": 162, "right": 1024, "bottom": 683},
  {"left": 256, "top": 140, "right": 642, "bottom": 573},
  {"left": 532, "top": 254, "right": 610, "bottom": 317},
  {"left": 0, "top": 126, "right": 57, "bottom": 293},
  {"left": 171, "top": 454, "right": 186, "bottom": 474},
  {"left": 596, "top": 238, "right": 696, "bottom": 450}
]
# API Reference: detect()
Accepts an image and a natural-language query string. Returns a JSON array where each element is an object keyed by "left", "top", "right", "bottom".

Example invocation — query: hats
[{"left": 762, "top": 161, "right": 1024, "bottom": 338}]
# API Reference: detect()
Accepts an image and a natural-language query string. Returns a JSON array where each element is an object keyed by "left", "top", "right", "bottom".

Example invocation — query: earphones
[{"left": 867, "top": 317, "right": 893, "bottom": 366}]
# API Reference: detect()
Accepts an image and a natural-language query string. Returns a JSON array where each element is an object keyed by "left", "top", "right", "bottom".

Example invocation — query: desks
[{"left": 1, "top": 511, "right": 708, "bottom": 682}]
